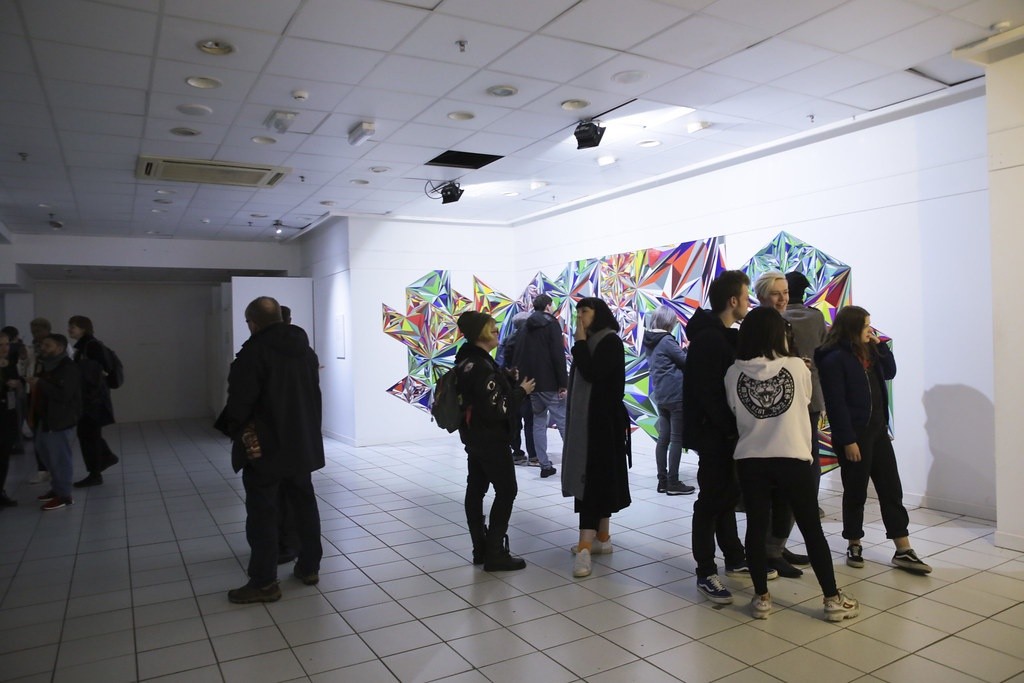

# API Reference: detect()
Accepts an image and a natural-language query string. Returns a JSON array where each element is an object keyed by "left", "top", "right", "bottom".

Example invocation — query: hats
[
  {"left": 457, "top": 310, "right": 489, "bottom": 344},
  {"left": 786, "top": 271, "right": 810, "bottom": 299}
]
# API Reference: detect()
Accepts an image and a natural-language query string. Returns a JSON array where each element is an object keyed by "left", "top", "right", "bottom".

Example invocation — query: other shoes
[
  {"left": 97, "top": 453, "right": 119, "bottom": 474},
  {"left": 781, "top": 548, "right": 812, "bottom": 568},
  {"left": 294, "top": 561, "right": 318, "bottom": 584},
  {"left": 512, "top": 454, "right": 528, "bottom": 464},
  {"left": 42, "top": 497, "right": 75, "bottom": 510},
  {"left": 571, "top": 535, "right": 612, "bottom": 554},
  {"left": 657, "top": 479, "right": 668, "bottom": 493},
  {"left": 667, "top": 482, "right": 695, "bottom": 494},
  {"left": 528, "top": 456, "right": 541, "bottom": 467},
  {"left": 10, "top": 446, "right": 25, "bottom": 456},
  {"left": 541, "top": 466, "right": 556, "bottom": 478},
  {"left": 29, "top": 473, "right": 51, "bottom": 484},
  {"left": 228, "top": 580, "right": 281, "bottom": 604},
  {"left": 573, "top": 548, "right": 591, "bottom": 577},
  {"left": 467, "top": 515, "right": 488, "bottom": 564},
  {"left": 0, "top": 491, "right": 17, "bottom": 508},
  {"left": 773, "top": 556, "right": 804, "bottom": 578},
  {"left": 38, "top": 490, "right": 58, "bottom": 502},
  {"left": 73, "top": 473, "right": 103, "bottom": 487}
]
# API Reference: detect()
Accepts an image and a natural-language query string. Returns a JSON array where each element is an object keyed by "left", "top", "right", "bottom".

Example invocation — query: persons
[
  {"left": 684, "top": 269, "right": 749, "bottom": 603},
  {"left": 754, "top": 271, "right": 809, "bottom": 578},
  {"left": 16, "top": 317, "right": 75, "bottom": 485},
  {"left": 814, "top": 306, "right": 933, "bottom": 573},
  {"left": 497, "top": 294, "right": 569, "bottom": 478},
  {"left": 0, "top": 326, "right": 30, "bottom": 512},
  {"left": 454, "top": 311, "right": 536, "bottom": 572},
  {"left": 213, "top": 297, "right": 325, "bottom": 603},
  {"left": 779, "top": 271, "right": 827, "bottom": 518},
  {"left": 724, "top": 307, "right": 859, "bottom": 621},
  {"left": 28, "top": 333, "right": 84, "bottom": 510},
  {"left": 642, "top": 306, "right": 695, "bottom": 496},
  {"left": 560, "top": 297, "right": 632, "bottom": 579},
  {"left": 69, "top": 316, "right": 119, "bottom": 489}
]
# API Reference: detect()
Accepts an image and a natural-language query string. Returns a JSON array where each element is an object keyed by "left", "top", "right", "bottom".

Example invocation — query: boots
[{"left": 485, "top": 523, "right": 526, "bottom": 572}]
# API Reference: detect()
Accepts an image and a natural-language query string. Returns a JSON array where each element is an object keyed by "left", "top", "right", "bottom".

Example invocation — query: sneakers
[
  {"left": 847, "top": 543, "right": 864, "bottom": 567},
  {"left": 823, "top": 589, "right": 859, "bottom": 622},
  {"left": 696, "top": 573, "right": 733, "bottom": 604},
  {"left": 750, "top": 592, "right": 772, "bottom": 619},
  {"left": 891, "top": 549, "right": 931, "bottom": 573},
  {"left": 724, "top": 557, "right": 778, "bottom": 580}
]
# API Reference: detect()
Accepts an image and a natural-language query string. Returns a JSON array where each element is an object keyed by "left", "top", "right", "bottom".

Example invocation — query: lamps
[
  {"left": 440, "top": 184, "right": 465, "bottom": 205},
  {"left": 574, "top": 119, "right": 607, "bottom": 150}
]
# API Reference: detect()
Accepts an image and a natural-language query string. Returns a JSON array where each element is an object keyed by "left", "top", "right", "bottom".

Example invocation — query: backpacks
[
  {"left": 431, "top": 355, "right": 494, "bottom": 432},
  {"left": 85, "top": 338, "right": 124, "bottom": 390}
]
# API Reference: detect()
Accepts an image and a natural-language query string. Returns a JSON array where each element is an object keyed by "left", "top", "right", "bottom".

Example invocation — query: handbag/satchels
[{"left": 495, "top": 334, "right": 515, "bottom": 368}]
